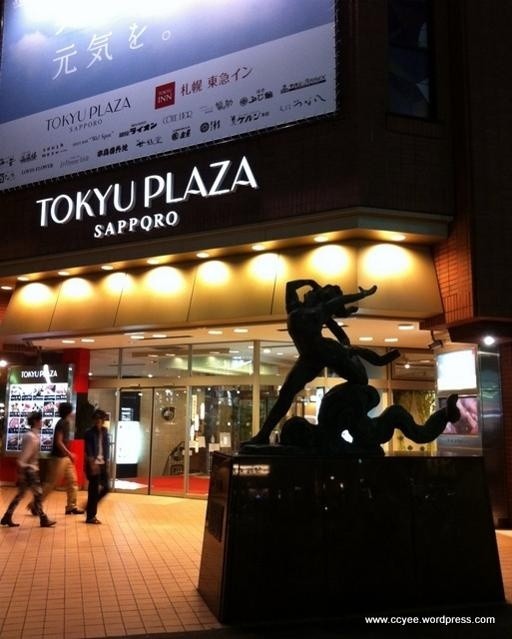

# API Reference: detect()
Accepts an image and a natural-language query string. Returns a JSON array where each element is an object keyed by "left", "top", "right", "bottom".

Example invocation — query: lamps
[{"left": 189, "top": 391, "right": 207, "bottom": 440}]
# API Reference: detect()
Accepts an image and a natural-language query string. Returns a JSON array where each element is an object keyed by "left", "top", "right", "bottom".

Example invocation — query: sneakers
[
  {"left": 25, "top": 502, "right": 38, "bottom": 516},
  {"left": 84, "top": 518, "right": 102, "bottom": 524},
  {"left": 65, "top": 505, "right": 85, "bottom": 515}
]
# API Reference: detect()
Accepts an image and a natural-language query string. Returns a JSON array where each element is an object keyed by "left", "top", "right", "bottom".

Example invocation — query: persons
[
  {"left": 81, "top": 409, "right": 112, "bottom": 524},
  {"left": 26, "top": 402, "right": 85, "bottom": 515},
  {"left": 240, "top": 277, "right": 377, "bottom": 447},
  {"left": 0, "top": 411, "right": 57, "bottom": 528}
]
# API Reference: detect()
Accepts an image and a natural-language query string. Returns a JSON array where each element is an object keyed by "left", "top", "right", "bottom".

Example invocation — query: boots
[
  {"left": 0, "top": 513, "right": 19, "bottom": 527},
  {"left": 39, "top": 514, "right": 56, "bottom": 528}
]
